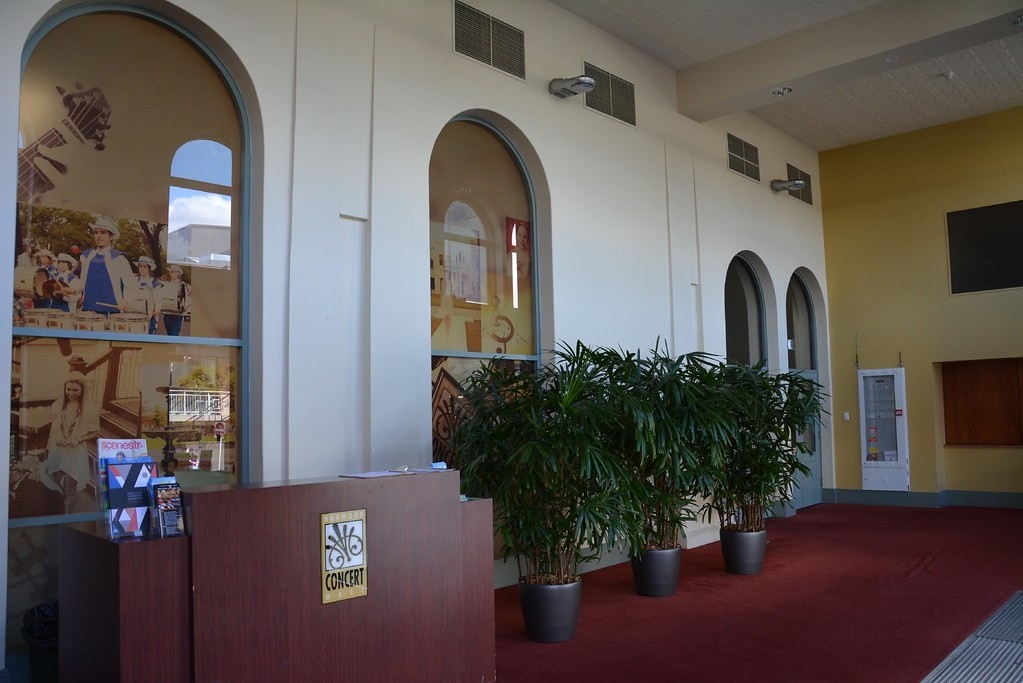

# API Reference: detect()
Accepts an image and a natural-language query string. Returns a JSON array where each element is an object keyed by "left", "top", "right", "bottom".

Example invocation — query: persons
[
  {"left": 32, "top": 250, "right": 57, "bottom": 308},
  {"left": 50, "top": 253, "right": 82, "bottom": 313},
  {"left": 134, "top": 256, "right": 163, "bottom": 335},
  {"left": 46, "top": 371, "right": 100, "bottom": 482},
  {"left": 78, "top": 218, "right": 137, "bottom": 317},
  {"left": 30, "top": 249, "right": 42, "bottom": 267},
  {"left": 161, "top": 265, "right": 191, "bottom": 336}
]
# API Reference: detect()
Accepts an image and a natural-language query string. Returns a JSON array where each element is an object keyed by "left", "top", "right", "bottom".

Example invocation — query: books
[{"left": 97, "top": 439, "right": 185, "bottom": 539}]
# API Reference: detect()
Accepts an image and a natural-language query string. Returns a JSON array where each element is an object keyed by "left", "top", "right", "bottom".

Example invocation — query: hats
[
  {"left": 167, "top": 264, "right": 183, "bottom": 277},
  {"left": 54, "top": 253, "right": 77, "bottom": 267},
  {"left": 38, "top": 250, "right": 55, "bottom": 262},
  {"left": 88, "top": 218, "right": 120, "bottom": 240},
  {"left": 134, "top": 256, "right": 157, "bottom": 271}
]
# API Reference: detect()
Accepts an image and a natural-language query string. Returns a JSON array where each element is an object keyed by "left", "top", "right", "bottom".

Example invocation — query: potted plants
[{"left": 447, "top": 333, "right": 832, "bottom": 644}]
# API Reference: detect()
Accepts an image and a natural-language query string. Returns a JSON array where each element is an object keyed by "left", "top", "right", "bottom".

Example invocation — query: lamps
[
  {"left": 771, "top": 179, "right": 805, "bottom": 192},
  {"left": 549, "top": 74, "right": 597, "bottom": 99}
]
